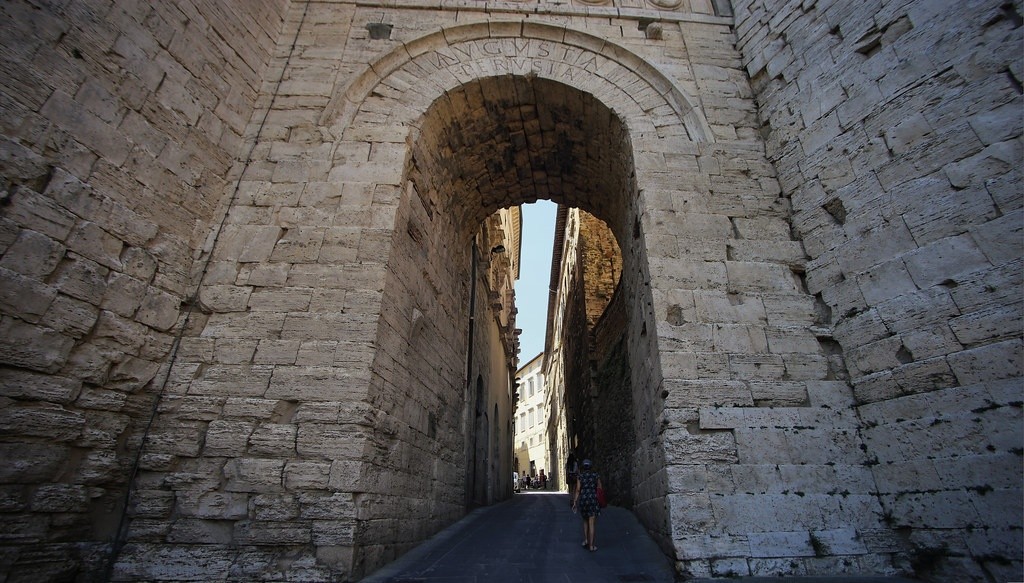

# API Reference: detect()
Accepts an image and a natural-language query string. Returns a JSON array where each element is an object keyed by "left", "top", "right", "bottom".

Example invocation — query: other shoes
[
  {"left": 590, "top": 544, "right": 599, "bottom": 551},
  {"left": 581, "top": 539, "right": 588, "bottom": 545}
]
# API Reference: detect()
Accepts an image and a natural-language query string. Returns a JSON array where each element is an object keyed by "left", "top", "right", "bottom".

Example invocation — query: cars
[{"left": 513, "top": 472, "right": 520, "bottom": 493}]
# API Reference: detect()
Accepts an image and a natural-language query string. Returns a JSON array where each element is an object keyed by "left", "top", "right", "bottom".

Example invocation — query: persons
[
  {"left": 522, "top": 474, "right": 527, "bottom": 489},
  {"left": 566, "top": 454, "right": 579, "bottom": 507},
  {"left": 526, "top": 474, "right": 531, "bottom": 489},
  {"left": 534, "top": 473, "right": 546, "bottom": 489},
  {"left": 573, "top": 458, "right": 602, "bottom": 552}
]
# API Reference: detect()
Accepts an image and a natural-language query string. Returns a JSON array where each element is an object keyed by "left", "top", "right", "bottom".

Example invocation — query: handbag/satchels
[{"left": 597, "top": 488, "right": 607, "bottom": 509}]
